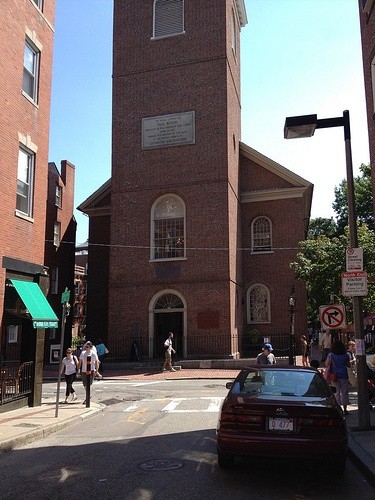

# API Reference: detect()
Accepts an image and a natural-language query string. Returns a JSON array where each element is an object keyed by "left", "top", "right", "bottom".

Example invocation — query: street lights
[
  {"left": 283, "top": 110, "right": 373, "bottom": 432},
  {"left": 287, "top": 287, "right": 298, "bottom": 365}
]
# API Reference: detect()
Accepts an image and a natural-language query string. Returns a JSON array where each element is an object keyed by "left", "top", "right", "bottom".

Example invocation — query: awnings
[{"left": 6, "top": 279, "right": 59, "bottom": 329}]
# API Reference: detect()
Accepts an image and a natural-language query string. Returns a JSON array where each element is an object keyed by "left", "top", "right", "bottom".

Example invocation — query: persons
[
  {"left": 321, "top": 330, "right": 331, "bottom": 361},
  {"left": 61, "top": 348, "right": 80, "bottom": 402},
  {"left": 301, "top": 335, "right": 311, "bottom": 367},
  {"left": 346, "top": 340, "right": 357, "bottom": 386},
  {"left": 365, "top": 328, "right": 375, "bottom": 403},
  {"left": 131, "top": 340, "right": 144, "bottom": 363},
  {"left": 325, "top": 339, "right": 351, "bottom": 416},
  {"left": 83, "top": 339, "right": 102, "bottom": 378},
  {"left": 96, "top": 340, "right": 105, "bottom": 373},
  {"left": 77, "top": 343, "right": 99, "bottom": 404},
  {"left": 310, "top": 361, "right": 329, "bottom": 385},
  {"left": 257, "top": 344, "right": 276, "bottom": 385},
  {"left": 162, "top": 332, "right": 176, "bottom": 372}
]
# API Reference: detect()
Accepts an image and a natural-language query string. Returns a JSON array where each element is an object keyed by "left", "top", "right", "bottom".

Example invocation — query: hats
[{"left": 264, "top": 343, "right": 273, "bottom": 350}]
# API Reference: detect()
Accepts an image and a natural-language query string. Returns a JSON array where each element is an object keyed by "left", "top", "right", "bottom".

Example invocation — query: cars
[{"left": 215, "top": 363, "right": 348, "bottom": 476}]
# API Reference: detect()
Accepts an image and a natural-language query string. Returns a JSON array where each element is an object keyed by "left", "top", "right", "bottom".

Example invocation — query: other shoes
[{"left": 344, "top": 410, "right": 350, "bottom": 416}]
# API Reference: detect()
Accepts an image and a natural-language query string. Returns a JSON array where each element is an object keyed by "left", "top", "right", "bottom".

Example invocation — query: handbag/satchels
[
  {"left": 329, "top": 373, "right": 336, "bottom": 381},
  {"left": 324, "top": 353, "right": 332, "bottom": 381}
]
